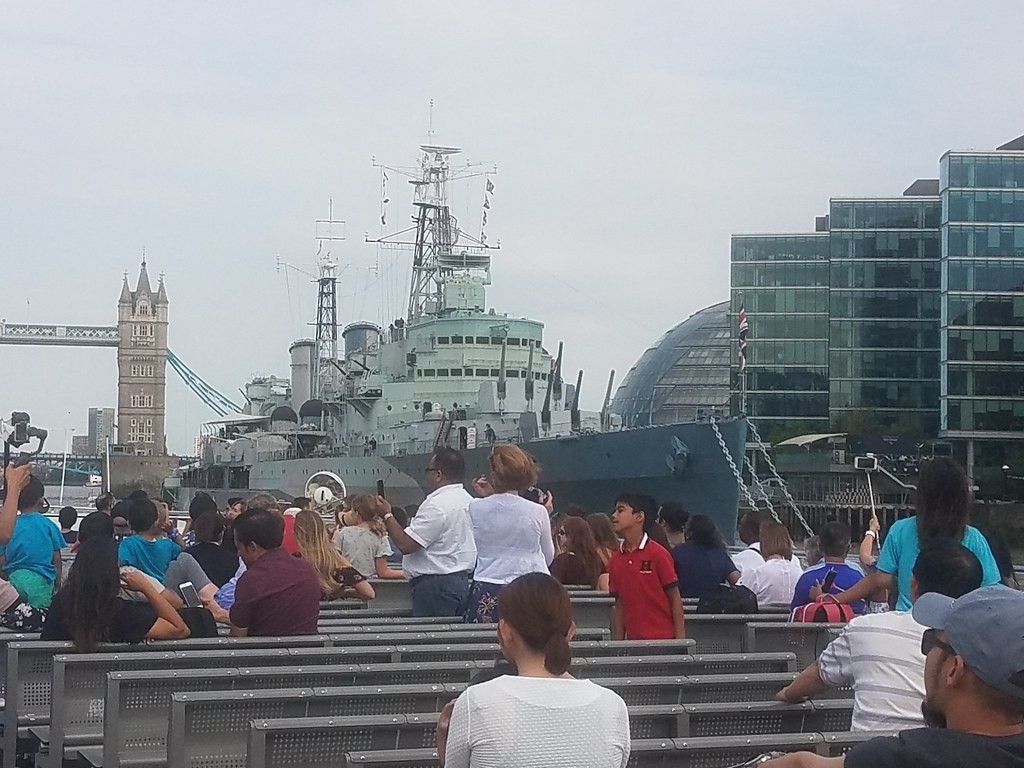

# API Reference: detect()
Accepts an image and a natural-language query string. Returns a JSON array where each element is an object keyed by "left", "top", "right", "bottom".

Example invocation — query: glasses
[
  {"left": 425, "top": 467, "right": 437, "bottom": 472},
  {"left": 921, "top": 629, "right": 955, "bottom": 656},
  {"left": 39, "top": 497, "right": 50, "bottom": 513},
  {"left": 559, "top": 529, "right": 566, "bottom": 535}
]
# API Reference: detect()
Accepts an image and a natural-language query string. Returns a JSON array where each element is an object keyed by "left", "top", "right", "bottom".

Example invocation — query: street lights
[{"left": 1002, "top": 465, "right": 1009, "bottom": 502}]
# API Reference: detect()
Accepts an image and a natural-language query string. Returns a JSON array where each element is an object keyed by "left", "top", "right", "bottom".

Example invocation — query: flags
[{"left": 739, "top": 304, "right": 750, "bottom": 377}]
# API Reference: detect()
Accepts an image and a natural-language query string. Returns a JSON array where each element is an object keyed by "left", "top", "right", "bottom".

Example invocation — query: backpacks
[
  {"left": 788, "top": 592, "right": 855, "bottom": 623},
  {"left": 697, "top": 583, "right": 758, "bottom": 614}
]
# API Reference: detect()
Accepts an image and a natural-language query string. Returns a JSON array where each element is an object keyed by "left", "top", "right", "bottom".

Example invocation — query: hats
[
  {"left": 338, "top": 510, "right": 353, "bottom": 526},
  {"left": 194, "top": 512, "right": 224, "bottom": 540},
  {"left": 912, "top": 584, "right": 1024, "bottom": 698}
]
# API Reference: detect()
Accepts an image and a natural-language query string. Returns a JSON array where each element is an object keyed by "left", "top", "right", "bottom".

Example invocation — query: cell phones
[
  {"left": 822, "top": 570, "right": 837, "bottom": 594},
  {"left": 377, "top": 479, "right": 385, "bottom": 503},
  {"left": 179, "top": 582, "right": 203, "bottom": 608},
  {"left": 854, "top": 457, "right": 877, "bottom": 470}
]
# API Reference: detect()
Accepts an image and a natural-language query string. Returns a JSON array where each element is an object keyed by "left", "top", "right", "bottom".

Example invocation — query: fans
[{"left": 305, "top": 472, "right": 347, "bottom": 518}]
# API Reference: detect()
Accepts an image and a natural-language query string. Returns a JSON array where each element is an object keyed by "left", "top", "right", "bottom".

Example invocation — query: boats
[
  {"left": 177, "top": 144, "right": 746, "bottom": 554},
  {"left": 85, "top": 475, "right": 103, "bottom": 487}
]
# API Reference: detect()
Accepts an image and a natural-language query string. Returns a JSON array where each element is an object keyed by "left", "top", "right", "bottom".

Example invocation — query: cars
[{"left": 761, "top": 478, "right": 787, "bottom": 486}]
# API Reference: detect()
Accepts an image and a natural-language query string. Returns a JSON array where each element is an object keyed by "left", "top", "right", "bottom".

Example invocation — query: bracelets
[
  {"left": 864, "top": 529, "right": 875, "bottom": 539},
  {"left": 384, "top": 512, "right": 393, "bottom": 521}
]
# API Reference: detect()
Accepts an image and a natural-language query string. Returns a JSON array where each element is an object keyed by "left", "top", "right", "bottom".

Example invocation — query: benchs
[{"left": 0, "top": 538, "right": 1024, "bottom": 768}]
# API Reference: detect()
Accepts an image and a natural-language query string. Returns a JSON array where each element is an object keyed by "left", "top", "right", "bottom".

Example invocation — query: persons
[
  {"left": 758, "top": 583, "right": 1024, "bottom": 767},
  {"left": 465, "top": 443, "right": 557, "bottom": 624},
  {"left": 607, "top": 492, "right": 685, "bottom": 640},
  {"left": 434, "top": 569, "right": 631, "bottom": 767},
  {"left": 860, "top": 516, "right": 902, "bottom": 607},
  {"left": 774, "top": 541, "right": 983, "bottom": 734},
  {"left": 670, "top": 513, "right": 742, "bottom": 598},
  {"left": 219, "top": 425, "right": 262, "bottom": 440},
  {"left": 805, "top": 534, "right": 865, "bottom": 577},
  {"left": 735, "top": 520, "right": 805, "bottom": 606},
  {"left": 731, "top": 510, "right": 803, "bottom": 576},
  {"left": 654, "top": 500, "right": 690, "bottom": 548},
  {"left": 363, "top": 435, "right": 377, "bottom": 458},
  {"left": 56, "top": 489, "right": 621, "bottom": 591},
  {"left": 41, "top": 535, "right": 192, "bottom": 642},
  {"left": 0, "top": 463, "right": 50, "bottom": 631},
  {"left": 483, "top": 423, "right": 497, "bottom": 445},
  {"left": 373, "top": 449, "right": 479, "bottom": 617},
  {"left": 200, "top": 509, "right": 321, "bottom": 639},
  {"left": 810, "top": 455, "right": 1005, "bottom": 611},
  {"left": 4, "top": 474, "right": 64, "bottom": 610},
  {"left": 791, "top": 521, "right": 867, "bottom": 613}
]
